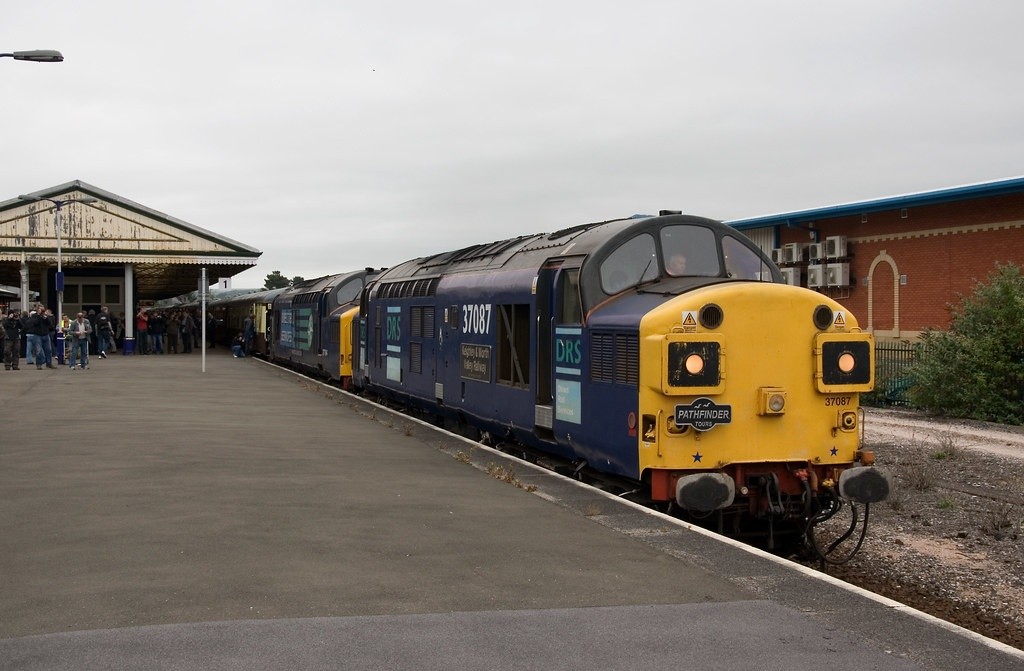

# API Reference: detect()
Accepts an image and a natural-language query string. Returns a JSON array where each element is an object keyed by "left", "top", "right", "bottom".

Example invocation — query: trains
[{"left": 135, "top": 209, "right": 888, "bottom": 569}]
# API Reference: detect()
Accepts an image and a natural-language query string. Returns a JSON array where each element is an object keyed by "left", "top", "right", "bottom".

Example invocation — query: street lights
[{"left": 18, "top": 195, "right": 98, "bottom": 364}]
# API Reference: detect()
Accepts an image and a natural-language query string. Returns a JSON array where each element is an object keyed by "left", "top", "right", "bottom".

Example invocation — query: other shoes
[
  {"left": 13, "top": 367, "right": 20, "bottom": 371},
  {"left": 81, "top": 366, "right": 89, "bottom": 369},
  {"left": 46, "top": 364, "right": 57, "bottom": 369},
  {"left": 71, "top": 366, "right": 75, "bottom": 370},
  {"left": 5, "top": 366, "right": 10, "bottom": 370},
  {"left": 37, "top": 365, "right": 42, "bottom": 370},
  {"left": 55, "top": 356, "right": 58, "bottom": 359},
  {"left": 27, "top": 362, "right": 34, "bottom": 364},
  {"left": 99, "top": 355, "right": 101, "bottom": 359},
  {"left": 232, "top": 354, "right": 237, "bottom": 357},
  {"left": 102, "top": 350, "right": 106, "bottom": 358}
]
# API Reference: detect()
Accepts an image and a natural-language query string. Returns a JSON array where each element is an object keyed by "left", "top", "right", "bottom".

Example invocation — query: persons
[
  {"left": 135, "top": 304, "right": 217, "bottom": 356},
  {"left": 231, "top": 313, "right": 256, "bottom": 358},
  {"left": 0, "top": 304, "right": 125, "bottom": 371}
]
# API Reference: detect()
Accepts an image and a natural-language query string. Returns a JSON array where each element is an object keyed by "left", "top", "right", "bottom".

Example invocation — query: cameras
[
  {"left": 13, "top": 313, "right": 18, "bottom": 318},
  {"left": 41, "top": 309, "right": 45, "bottom": 312}
]
